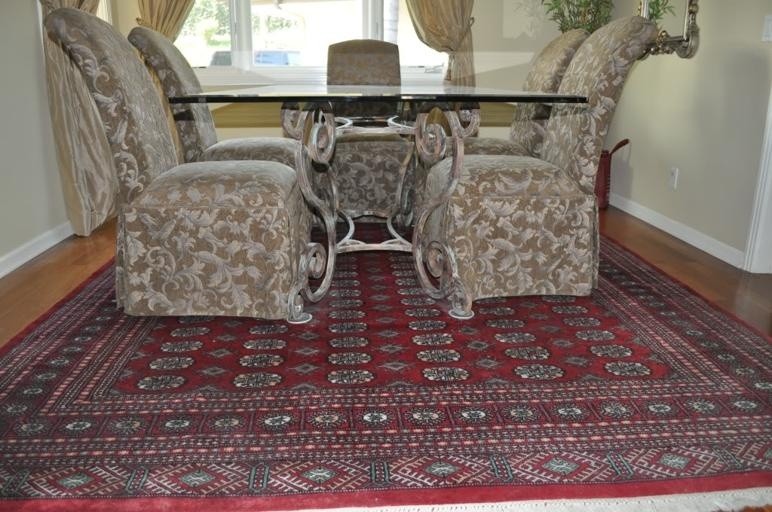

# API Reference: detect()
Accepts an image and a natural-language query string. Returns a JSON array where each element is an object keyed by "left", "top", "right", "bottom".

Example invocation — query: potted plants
[{"left": 540, "top": 0, "right": 613, "bottom": 34}]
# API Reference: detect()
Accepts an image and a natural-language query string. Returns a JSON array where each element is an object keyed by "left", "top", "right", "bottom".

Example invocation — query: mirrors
[{"left": 635, "top": 0, "right": 701, "bottom": 61}]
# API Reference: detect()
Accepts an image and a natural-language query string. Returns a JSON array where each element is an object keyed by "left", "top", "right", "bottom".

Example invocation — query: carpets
[{"left": 0, "top": 255, "right": 772, "bottom": 511}]
